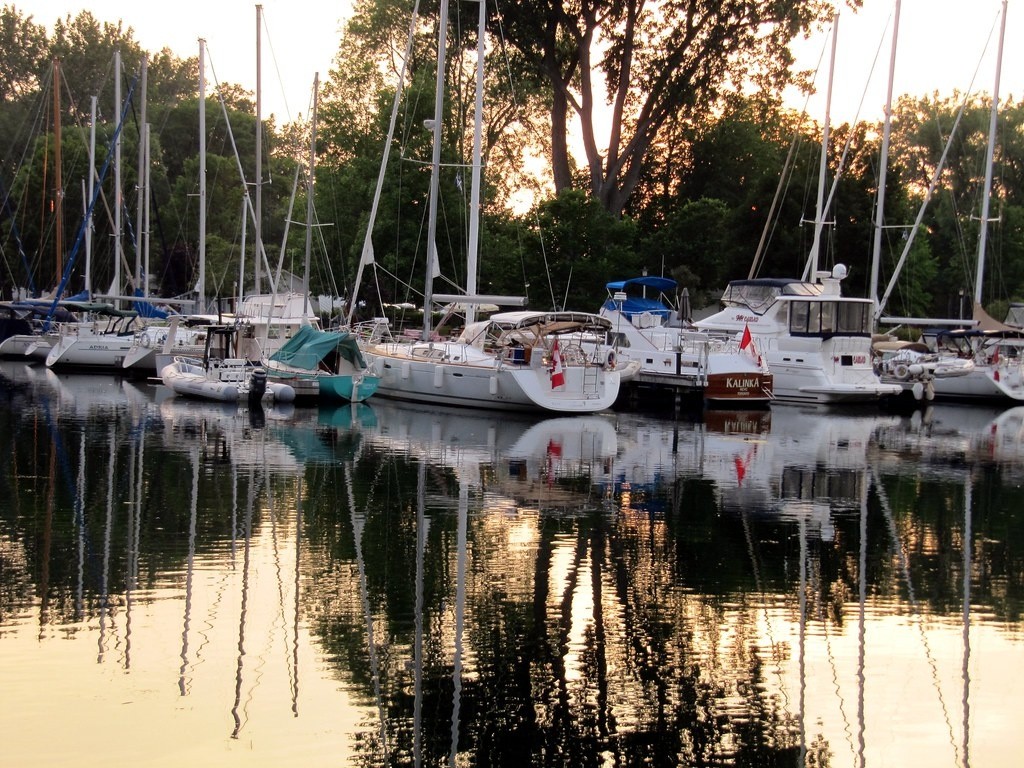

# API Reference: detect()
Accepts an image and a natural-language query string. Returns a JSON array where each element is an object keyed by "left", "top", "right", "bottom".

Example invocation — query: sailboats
[
  {"left": 4, "top": 395, "right": 1024, "bottom": 768},
  {"left": 0, "top": 0, "right": 1024, "bottom": 413}
]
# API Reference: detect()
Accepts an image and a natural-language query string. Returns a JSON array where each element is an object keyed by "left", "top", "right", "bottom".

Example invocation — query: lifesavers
[
  {"left": 141, "top": 333, "right": 150, "bottom": 347},
  {"left": 995, "top": 354, "right": 1005, "bottom": 368},
  {"left": 604, "top": 348, "right": 617, "bottom": 369},
  {"left": 895, "top": 365, "right": 908, "bottom": 379},
  {"left": 640, "top": 312, "right": 652, "bottom": 329}
]
